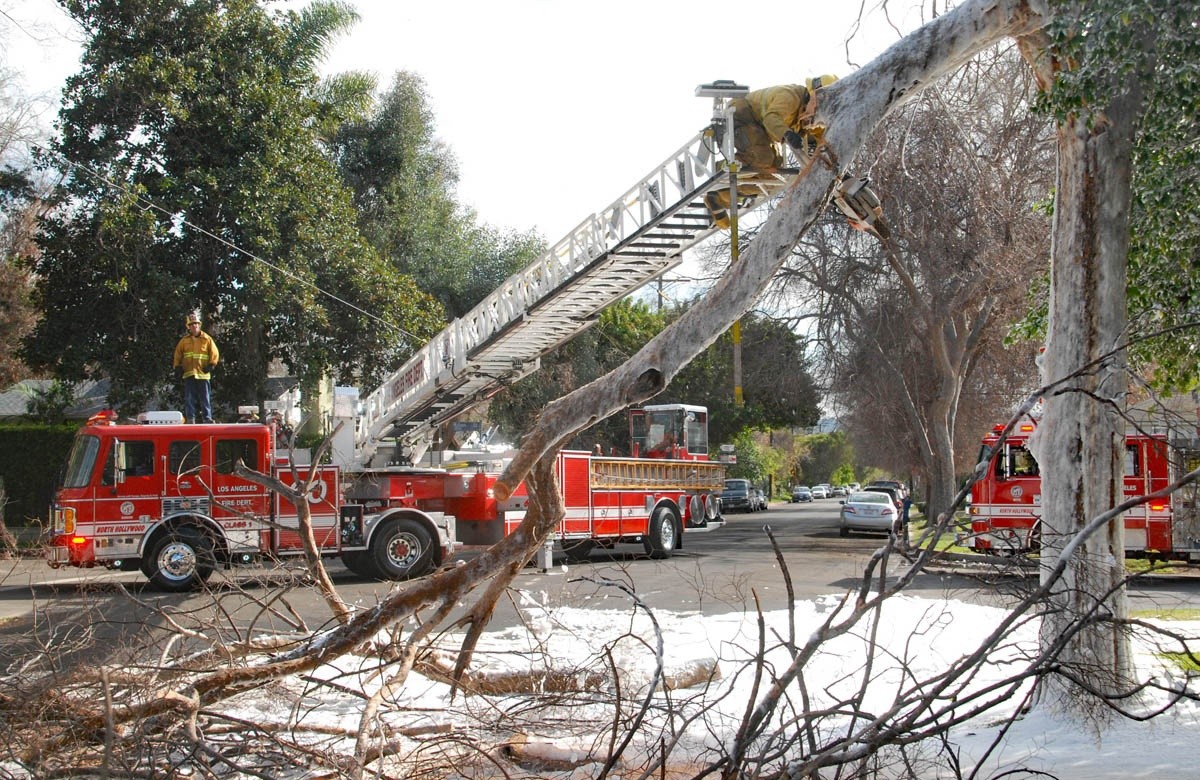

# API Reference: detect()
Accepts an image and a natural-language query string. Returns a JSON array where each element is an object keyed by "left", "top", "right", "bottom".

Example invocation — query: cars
[
  {"left": 790, "top": 479, "right": 911, "bottom": 531},
  {"left": 719, "top": 479, "right": 769, "bottom": 514},
  {"left": 838, "top": 491, "right": 901, "bottom": 538}
]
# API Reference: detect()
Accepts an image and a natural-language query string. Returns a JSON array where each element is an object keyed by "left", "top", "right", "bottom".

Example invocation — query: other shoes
[
  {"left": 186, "top": 418, "right": 195, "bottom": 424},
  {"left": 202, "top": 419, "right": 216, "bottom": 424},
  {"left": 704, "top": 191, "right": 731, "bottom": 229}
]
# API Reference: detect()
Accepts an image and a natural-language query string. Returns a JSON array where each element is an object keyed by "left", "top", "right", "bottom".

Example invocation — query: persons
[
  {"left": 174, "top": 315, "right": 220, "bottom": 423},
  {"left": 1016, "top": 452, "right": 1039, "bottom": 475},
  {"left": 590, "top": 443, "right": 604, "bottom": 457},
  {"left": 703, "top": 75, "right": 841, "bottom": 230}
]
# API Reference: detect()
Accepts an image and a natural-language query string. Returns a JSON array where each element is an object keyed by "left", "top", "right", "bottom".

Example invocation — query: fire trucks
[
  {"left": 952, "top": 419, "right": 1200, "bottom": 565},
  {"left": 40, "top": 78, "right": 802, "bottom": 595}
]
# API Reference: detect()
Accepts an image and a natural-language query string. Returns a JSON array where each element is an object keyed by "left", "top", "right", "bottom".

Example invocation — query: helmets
[
  {"left": 186, "top": 314, "right": 202, "bottom": 329},
  {"left": 806, "top": 75, "right": 838, "bottom": 94}
]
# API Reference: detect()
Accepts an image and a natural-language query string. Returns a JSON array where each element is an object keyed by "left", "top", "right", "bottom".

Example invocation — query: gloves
[
  {"left": 174, "top": 367, "right": 184, "bottom": 379},
  {"left": 205, "top": 365, "right": 215, "bottom": 373},
  {"left": 785, "top": 131, "right": 804, "bottom": 150}
]
